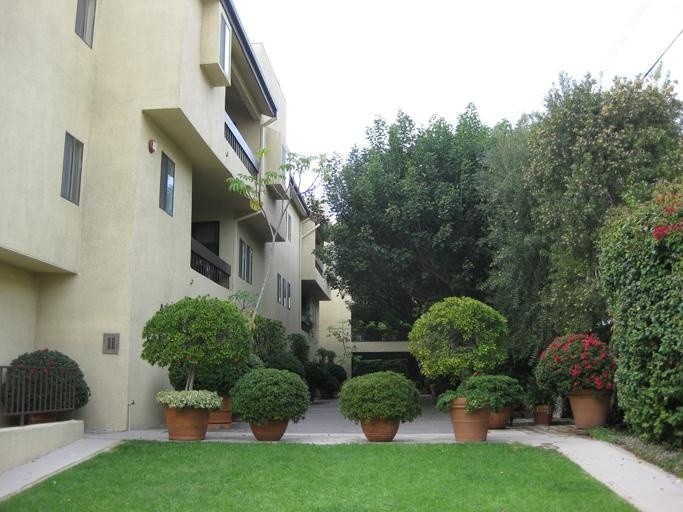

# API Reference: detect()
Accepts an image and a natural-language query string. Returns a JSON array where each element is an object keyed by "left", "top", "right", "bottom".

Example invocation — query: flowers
[{"left": 534, "top": 332, "right": 616, "bottom": 397}]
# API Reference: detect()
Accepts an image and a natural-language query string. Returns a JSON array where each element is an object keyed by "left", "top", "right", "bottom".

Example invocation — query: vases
[{"left": 567, "top": 389, "right": 611, "bottom": 429}]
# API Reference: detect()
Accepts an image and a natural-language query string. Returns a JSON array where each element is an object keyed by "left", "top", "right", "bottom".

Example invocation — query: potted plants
[
  {"left": 227, "top": 365, "right": 311, "bottom": 442},
  {"left": 156, "top": 387, "right": 225, "bottom": 443},
  {"left": 458, "top": 374, "right": 555, "bottom": 430},
  {"left": 435, "top": 385, "right": 504, "bottom": 443},
  {"left": 167, "top": 346, "right": 350, "bottom": 429},
  {"left": 4, "top": 348, "right": 91, "bottom": 425},
  {"left": 337, "top": 368, "right": 428, "bottom": 443}
]
